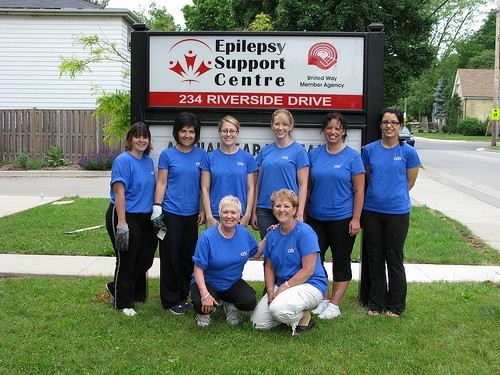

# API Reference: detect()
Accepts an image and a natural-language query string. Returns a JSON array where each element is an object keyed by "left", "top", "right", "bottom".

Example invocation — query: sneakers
[
  {"left": 311, "top": 302, "right": 328, "bottom": 314},
  {"left": 318, "top": 308, "right": 341, "bottom": 319},
  {"left": 223, "top": 304, "right": 242, "bottom": 325},
  {"left": 195, "top": 314, "right": 212, "bottom": 327}
]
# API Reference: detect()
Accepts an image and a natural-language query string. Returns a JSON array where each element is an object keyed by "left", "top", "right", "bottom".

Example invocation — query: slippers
[{"left": 296, "top": 319, "right": 314, "bottom": 333}]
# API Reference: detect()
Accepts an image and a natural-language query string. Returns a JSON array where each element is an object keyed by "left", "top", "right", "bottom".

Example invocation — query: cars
[{"left": 398, "top": 126, "right": 415, "bottom": 147}]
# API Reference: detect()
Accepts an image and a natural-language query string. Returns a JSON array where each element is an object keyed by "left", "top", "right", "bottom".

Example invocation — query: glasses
[
  {"left": 381, "top": 121, "right": 401, "bottom": 125},
  {"left": 220, "top": 130, "right": 237, "bottom": 135}
]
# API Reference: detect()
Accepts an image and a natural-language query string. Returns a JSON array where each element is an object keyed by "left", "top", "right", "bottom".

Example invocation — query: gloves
[
  {"left": 115, "top": 224, "right": 129, "bottom": 251},
  {"left": 150, "top": 202, "right": 167, "bottom": 239}
]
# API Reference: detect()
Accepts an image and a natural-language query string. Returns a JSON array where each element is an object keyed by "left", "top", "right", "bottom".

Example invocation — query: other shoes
[
  {"left": 168, "top": 303, "right": 184, "bottom": 316},
  {"left": 386, "top": 310, "right": 399, "bottom": 318},
  {"left": 182, "top": 301, "right": 193, "bottom": 308},
  {"left": 367, "top": 309, "right": 378, "bottom": 315},
  {"left": 123, "top": 307, "right": 137, "bottom": 316},
  {"left": 106, "top": 284, "right": 114, "bottom": 301}
]
